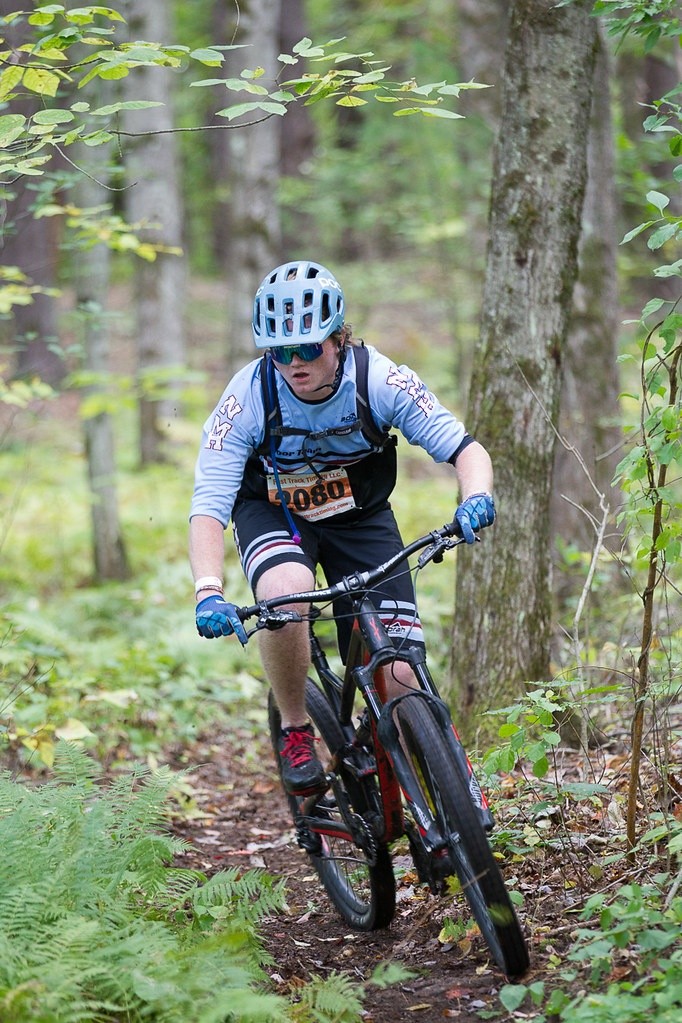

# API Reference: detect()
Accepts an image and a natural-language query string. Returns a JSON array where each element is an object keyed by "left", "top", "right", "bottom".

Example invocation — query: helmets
[{"left": 251, "top": 261, "right": 345, "bottom": 348}]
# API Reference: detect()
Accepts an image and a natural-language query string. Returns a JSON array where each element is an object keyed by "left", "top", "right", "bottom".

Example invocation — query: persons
[{"left": 189, "top": 258, "right": 497, "bottom": 884}]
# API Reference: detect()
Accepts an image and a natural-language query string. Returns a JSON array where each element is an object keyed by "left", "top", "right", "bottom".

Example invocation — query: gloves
[
  {"left": 195, "top": 594, "right": 248, "bottom": 644},
  {"left": 452, "top": 492, "right": 496, "bottom": 544}
]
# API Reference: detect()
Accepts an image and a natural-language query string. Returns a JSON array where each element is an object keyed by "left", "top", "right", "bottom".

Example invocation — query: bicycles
[{"left": 235, "top": 516, "right": 534, "bottom": 979}]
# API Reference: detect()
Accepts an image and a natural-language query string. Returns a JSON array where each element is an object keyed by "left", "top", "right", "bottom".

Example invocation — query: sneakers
[
  {"left": 415, "top": 848, "right": 455, "bottom": 882},
  {"left": 277, "top": 724, "right": 328, "bottom": 796}
]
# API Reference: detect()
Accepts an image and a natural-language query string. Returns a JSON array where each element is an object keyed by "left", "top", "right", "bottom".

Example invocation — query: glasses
[{"left": 270, "top": 343, "right": 323, "bottom": 364}]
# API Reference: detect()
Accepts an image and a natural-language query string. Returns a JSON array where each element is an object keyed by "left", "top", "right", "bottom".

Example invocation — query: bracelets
[{"left": 195, "top": 577, "right": 223, "bottom": 599}]
